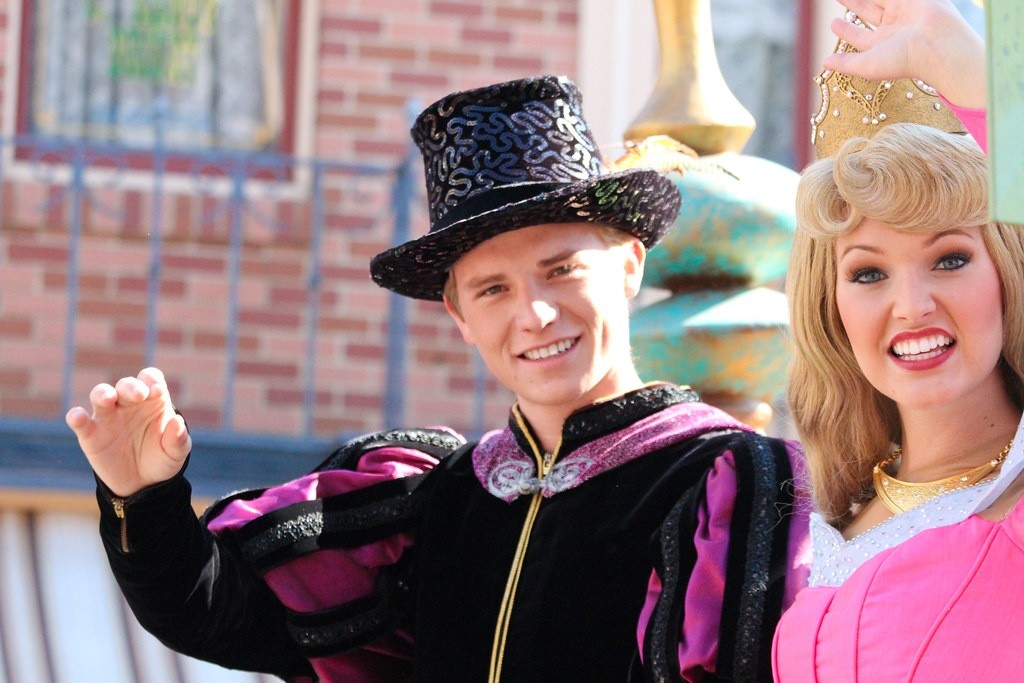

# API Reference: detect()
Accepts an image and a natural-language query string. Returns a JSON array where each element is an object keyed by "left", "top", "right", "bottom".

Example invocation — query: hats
[{"left": 368, "top": 75, "right": 683, "bottom": 301}]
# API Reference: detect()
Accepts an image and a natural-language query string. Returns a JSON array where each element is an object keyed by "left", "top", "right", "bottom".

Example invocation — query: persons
[
  {"left": 66, "top": 74, "right": 809, "bottom": 683},
  {"left": 770, "top": 0, "right": 1024, "bottom": 683}
]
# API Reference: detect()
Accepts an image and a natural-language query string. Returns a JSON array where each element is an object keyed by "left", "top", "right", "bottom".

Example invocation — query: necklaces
[{"left": 873, "top": 440, "right": 1014, "bottom": 515}]
